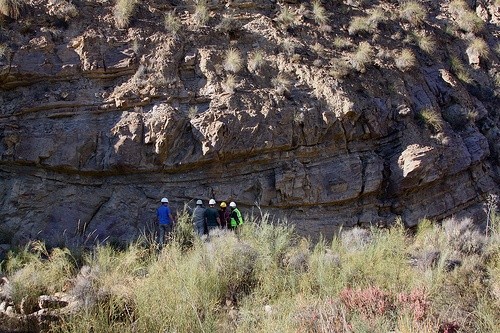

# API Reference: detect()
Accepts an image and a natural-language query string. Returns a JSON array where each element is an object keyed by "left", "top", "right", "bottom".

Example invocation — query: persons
[
  {"left": 189, "top": 200, "right": 205, "bottom": 235},
  {"left": 156, "top": 197, "right": 175, "bottom": 247},
  {"left": 229, "top": 202, "right": 243, "bottom": 230},
  {"left": 204, "top": 199, "right": 221, "bottom": 232},
  {"left": 219, "top": 202, "right": 229, "bottom": 229}
]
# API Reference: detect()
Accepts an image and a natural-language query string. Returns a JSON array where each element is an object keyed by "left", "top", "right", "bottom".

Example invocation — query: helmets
[
  {"left": 196, "top": 200, "right": 202, "bottom": 204},
  {"left": 219, "top": 202, "right": 227, "bottom": 208},
  {"left": 209, "top": 199, "right": 216, "bottom": 205},
  {"left": 161, "top": 198, "right": 169, "bottom": 203},
  {"left": 229, "top": 202, "right": 236, "bottom": 207}
]
